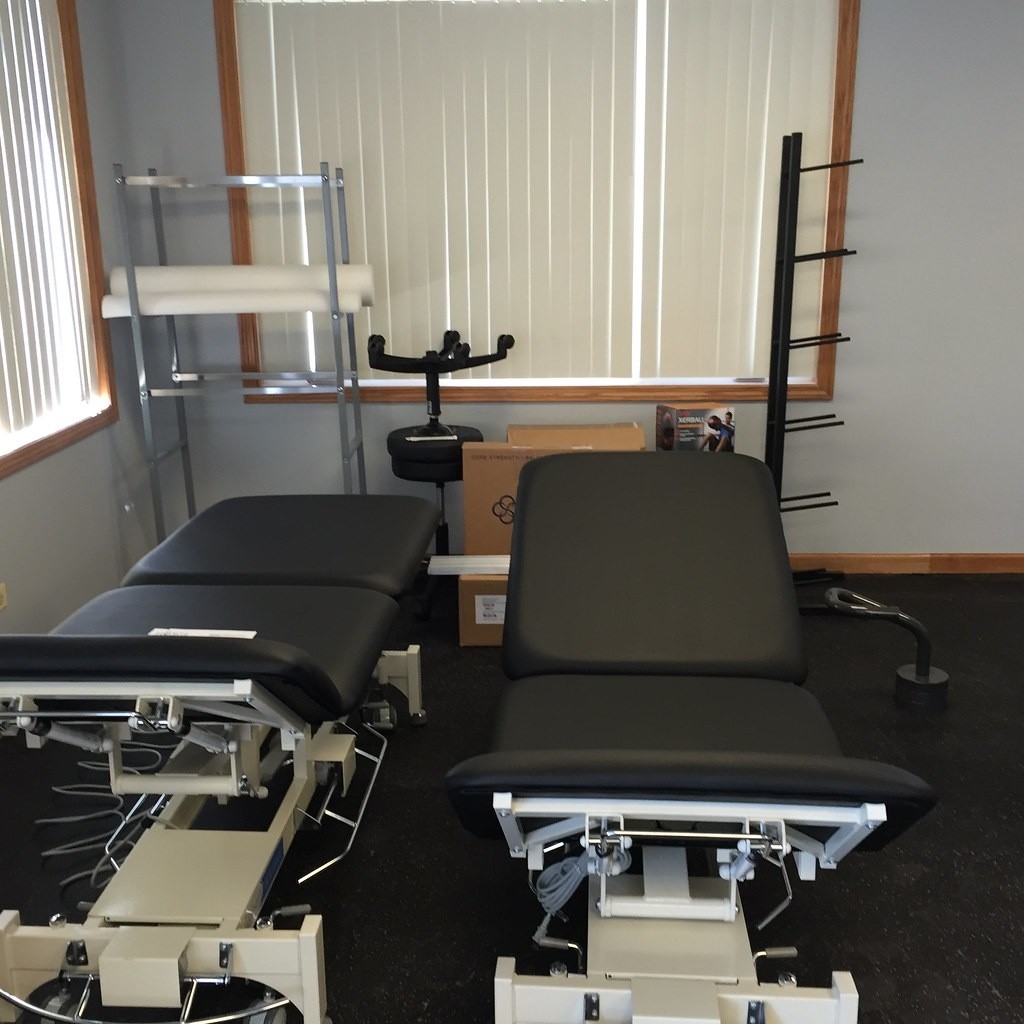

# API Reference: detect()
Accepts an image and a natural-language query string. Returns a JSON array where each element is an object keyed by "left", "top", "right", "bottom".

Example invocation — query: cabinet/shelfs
[{"left": 112, "top": 162, "right": 366, "bottom": 542}]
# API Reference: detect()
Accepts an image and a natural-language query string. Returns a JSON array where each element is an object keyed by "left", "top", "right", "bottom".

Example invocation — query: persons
[{"left": 699, "top": 412, "right": 734, "bottom": 452}]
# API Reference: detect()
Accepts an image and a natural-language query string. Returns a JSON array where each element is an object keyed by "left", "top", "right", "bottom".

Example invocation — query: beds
[
  {"left": 446, "top": 449, "right": 936, "bottom": 1024},
  {"left": 0, "top": 494, "right": 438, "bottom": 1023}
]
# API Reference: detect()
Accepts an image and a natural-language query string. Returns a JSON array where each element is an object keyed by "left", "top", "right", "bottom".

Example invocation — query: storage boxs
[{"left": 460, "top": 421, "right": 645, "bottom": 645}]
